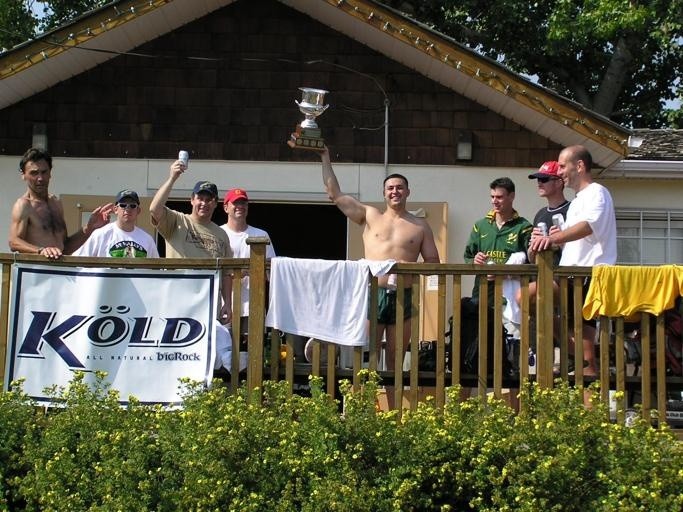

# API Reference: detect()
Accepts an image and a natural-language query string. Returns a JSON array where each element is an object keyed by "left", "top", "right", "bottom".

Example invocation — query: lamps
[
  {"left": 29, "top": 122, "right": 49, "bottom": 154},
  {"left": 455, "top": 130, "right": 477, "bottom": 162}
]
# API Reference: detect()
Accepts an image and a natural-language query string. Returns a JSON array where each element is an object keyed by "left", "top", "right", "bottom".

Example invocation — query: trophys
[{"left": 286, "top": 85, "right": 330, "bottom": 151}]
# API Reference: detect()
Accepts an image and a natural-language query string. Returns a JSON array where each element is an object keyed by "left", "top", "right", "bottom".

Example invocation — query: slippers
[{"left": 553, "top": 354, "right": 587, "bottom": 378}]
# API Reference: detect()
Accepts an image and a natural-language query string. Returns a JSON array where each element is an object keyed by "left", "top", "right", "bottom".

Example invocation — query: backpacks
[{"left": 448, "top": 297, "right": 512, "bottom": 378}]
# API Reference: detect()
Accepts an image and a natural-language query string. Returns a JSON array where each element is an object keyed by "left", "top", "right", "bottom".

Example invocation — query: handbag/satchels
[{"left": 418, "top": 340, "right": 438, "bottom": 371}]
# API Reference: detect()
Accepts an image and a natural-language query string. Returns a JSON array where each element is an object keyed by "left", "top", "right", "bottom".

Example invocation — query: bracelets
[{"left": 37, "top": 247, "right": 44, "bottom": 254}]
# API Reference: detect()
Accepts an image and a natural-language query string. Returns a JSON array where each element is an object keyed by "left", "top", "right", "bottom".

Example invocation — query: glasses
[
  {"left": 536, "top": 178, "right": 549, "bottom": 183},
  {"left": 117, "top": 204, "right": 136, "bottom": 208}
]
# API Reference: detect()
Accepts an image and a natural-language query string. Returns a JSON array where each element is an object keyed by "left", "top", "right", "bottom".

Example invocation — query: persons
[
  {"left": 315, "top": 141, "right": 440, "bottom": 411},
  {"left": 514, "top": 145, "right": 617, "bottom": 410},
  {"left": 7, "top": 146, "right": 114, "bottom": 260},
  {"left": 459, "top": 176, "right": 532, "bottom": 412},
  {"left": 526, "top": 159, "right": 572, "bottom": 266},
  {"left": 217, "top": 187, "right": 276, "bottom": 353},
  {"left": 148, "top": 159, "right": 234, "bottom": 324},
  {"left": 69, "top": 189, "right": 162, "bottom": 259}
]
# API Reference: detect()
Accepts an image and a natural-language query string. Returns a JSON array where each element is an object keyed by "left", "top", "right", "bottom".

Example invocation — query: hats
[
  {"left": 528, "top": 161, "right": 563, "bottom": 180},
  {"left": 222, "top": 190, "right": 249, "bottom": 205},
  {"left": 192, "top": 181, "right": 218, "bottom": 198},
  {"left": 114, "top": 190, "right": 139, "bottom": 204}
]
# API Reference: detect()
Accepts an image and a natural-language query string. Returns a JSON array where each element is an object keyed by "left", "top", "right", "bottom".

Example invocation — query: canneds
[
  {"left": 537, "top": 222, "right": 547, "bottom": 238},
  {"left": 178, "top": 150, "right": 188, "bottom": 169},
  {"left": 552, "top": 213, "right": 564, "bottom": 226}
]
